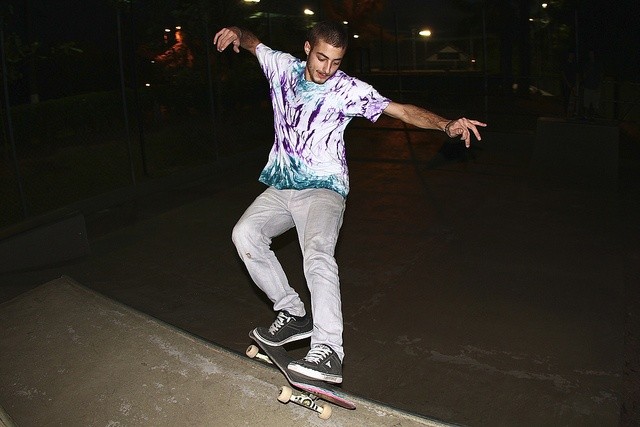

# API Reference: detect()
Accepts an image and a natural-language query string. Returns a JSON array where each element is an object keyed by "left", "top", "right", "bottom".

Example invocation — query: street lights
[
  {"left": 303, "top": 8, "right": 314, "bottom": 41},
  {"left": 542, "top": 2, "right": 581, "bottom": 118},
  {"left": 419, "top": 30, "right": 431, "bottom": 69},
  {"left": 353, "top": 34, "right": 362, "bottom": 74},
  {"left": 245, "top": 0, "right": 273, "bottom": 41}
]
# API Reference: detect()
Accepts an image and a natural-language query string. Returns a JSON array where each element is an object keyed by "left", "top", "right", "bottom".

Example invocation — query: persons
[{"left": 211, "top": 21, "right": 487, "bottom": 384}]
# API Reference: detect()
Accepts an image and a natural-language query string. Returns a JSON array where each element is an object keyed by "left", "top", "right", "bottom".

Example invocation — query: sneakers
[
  {"left": 287, "top": 345, "right": 343, "bottom": 386},
  {"left": 253, "top": 311, "right": 313, "bottom": 348}
]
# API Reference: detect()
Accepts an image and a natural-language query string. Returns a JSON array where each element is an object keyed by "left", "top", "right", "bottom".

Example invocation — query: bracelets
[{"left": 446, "top": 115, "right": 454, "bottom": 141}]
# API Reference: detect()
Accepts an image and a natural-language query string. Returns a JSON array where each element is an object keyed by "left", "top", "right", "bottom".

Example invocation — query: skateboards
[{"left": 246, "top": 329, "right": 356, "bottom": 419}]
